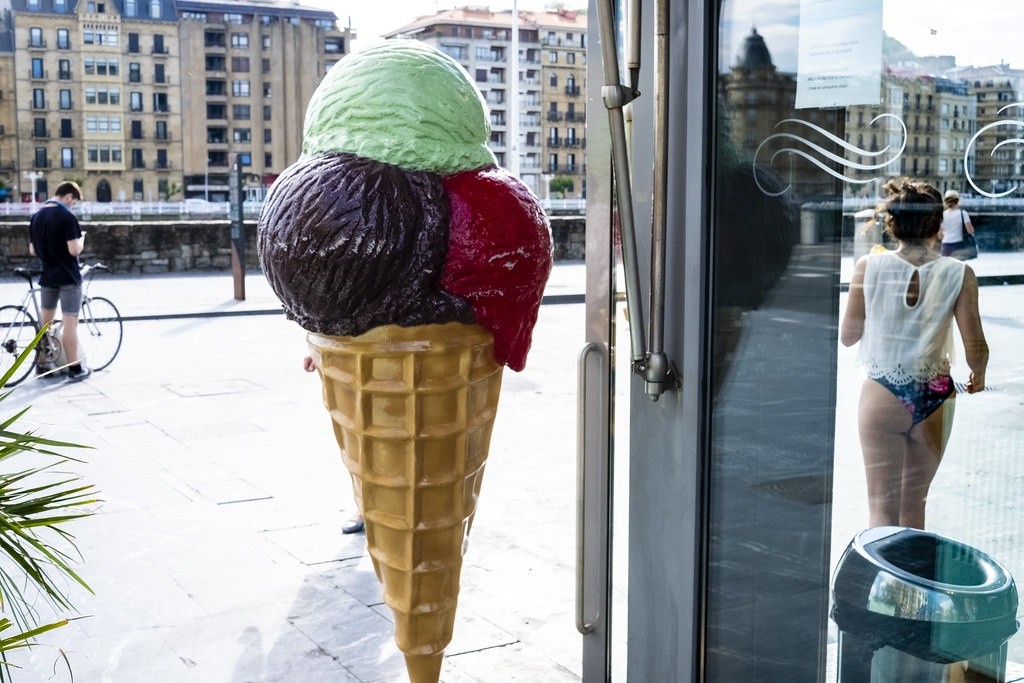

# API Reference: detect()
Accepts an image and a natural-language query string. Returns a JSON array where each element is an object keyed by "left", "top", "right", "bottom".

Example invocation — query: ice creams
[{"left": 257, "top": 38, "right": 554, "bottom": 683}]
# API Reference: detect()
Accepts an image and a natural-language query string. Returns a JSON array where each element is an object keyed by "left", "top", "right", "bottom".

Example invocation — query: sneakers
[
  {"left": 35, "top": 366, "right": 60, "bottom": 378},
  {"left": 68, "top": 364, "right": 90, "bottom": 381}
]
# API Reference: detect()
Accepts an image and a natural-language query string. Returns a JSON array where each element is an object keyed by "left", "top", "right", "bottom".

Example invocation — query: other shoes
[{"left": 341, "top": 515, "right": 364, "bottom": 534}]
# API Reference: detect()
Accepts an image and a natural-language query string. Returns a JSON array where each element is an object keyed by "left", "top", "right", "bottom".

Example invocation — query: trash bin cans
[
  {"left": 854, "top": 209, "right": 898, "bottom": 269},
  {"left": 826, "top": 524, "right": 1023, "bottom": 683}
]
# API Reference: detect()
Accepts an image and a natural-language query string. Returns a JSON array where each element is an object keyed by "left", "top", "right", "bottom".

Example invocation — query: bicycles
[{"left": 0, "top": 262, "right": 123, "bottom": 387}]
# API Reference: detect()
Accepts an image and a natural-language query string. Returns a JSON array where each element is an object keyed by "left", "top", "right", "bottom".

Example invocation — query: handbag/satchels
[{"left": 963, "top": 231, "right": 977, "bottom": 259}]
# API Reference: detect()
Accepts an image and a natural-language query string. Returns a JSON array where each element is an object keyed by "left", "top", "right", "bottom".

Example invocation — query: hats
[{"left": 943, "top": 189, "right": 960, "bottom": 201}]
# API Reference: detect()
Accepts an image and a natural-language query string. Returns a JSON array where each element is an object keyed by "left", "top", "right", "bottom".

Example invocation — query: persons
[
  {"left": 838, "top": 176, "right": 988, "bottom": 534},
  {"left": 27, "top": 180, "right": 94, "bottom": 382},
  {"left": 934, "top": 187, "right": 975, "bottom": 262}
]
